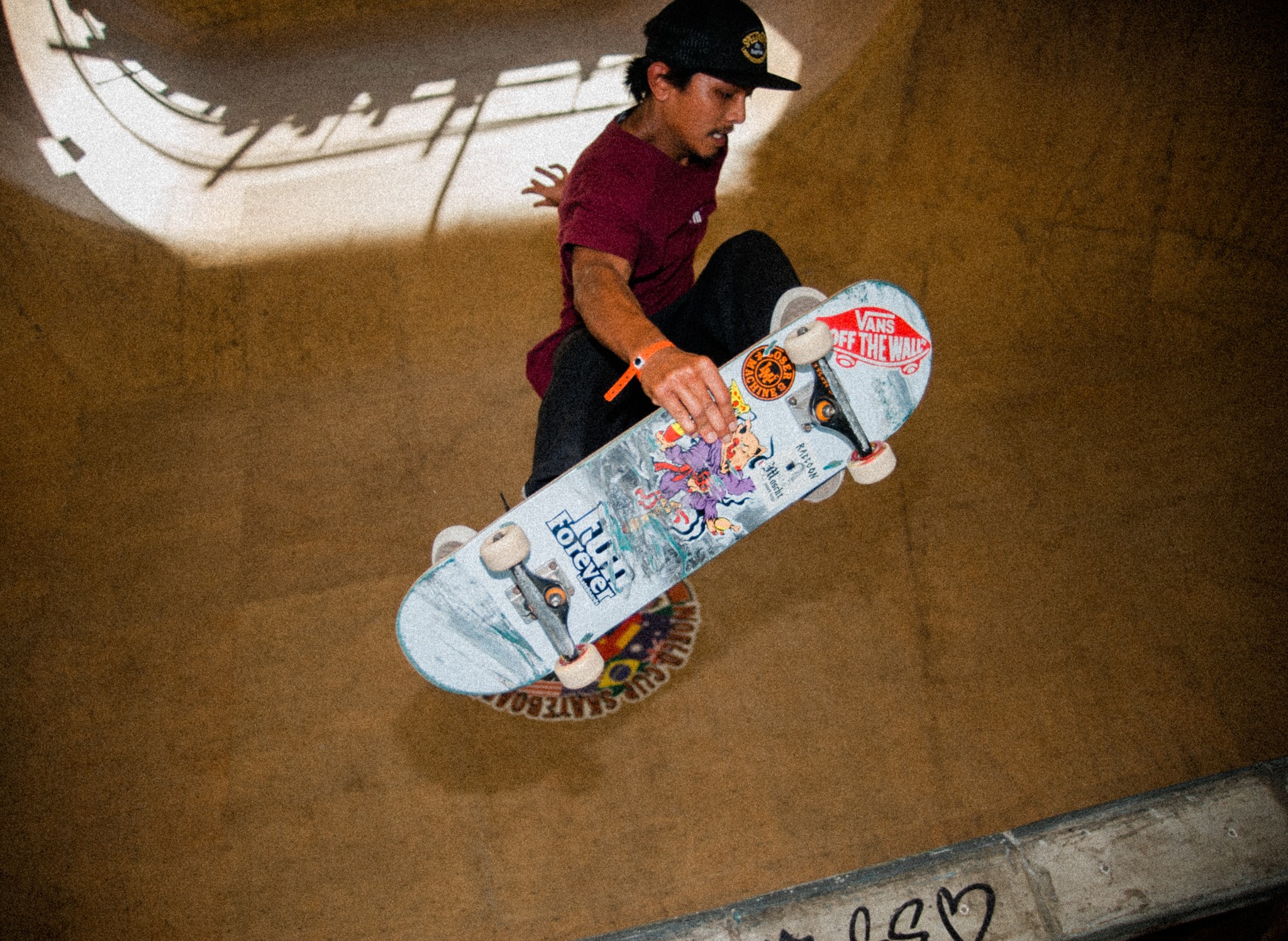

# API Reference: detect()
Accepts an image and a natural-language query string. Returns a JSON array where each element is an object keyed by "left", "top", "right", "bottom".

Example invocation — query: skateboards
[{"left": 393, "top": 277, "right": 935, "bottom": 706}]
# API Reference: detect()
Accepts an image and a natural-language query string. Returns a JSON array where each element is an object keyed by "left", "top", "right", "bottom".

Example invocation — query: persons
[{"left": 429, "top": 0, "right": 846, "bottom": 568}]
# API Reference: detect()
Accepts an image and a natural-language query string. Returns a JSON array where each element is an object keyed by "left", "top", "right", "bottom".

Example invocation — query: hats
[{"left": 643, "top": 0, "right": 803, "bottom": 93}]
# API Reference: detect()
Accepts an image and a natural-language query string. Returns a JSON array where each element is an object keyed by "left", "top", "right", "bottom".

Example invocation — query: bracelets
[{"left": 605, "top": 339, "right": 677, "bottom": 403}]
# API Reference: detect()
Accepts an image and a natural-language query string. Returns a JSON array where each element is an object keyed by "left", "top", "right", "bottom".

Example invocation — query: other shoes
[
  {"left": 429, "top": 523, "right": 476, "bottom": 565},
  {"left": 770, "top": 287, "right": 848, "bottom": 504}
]
